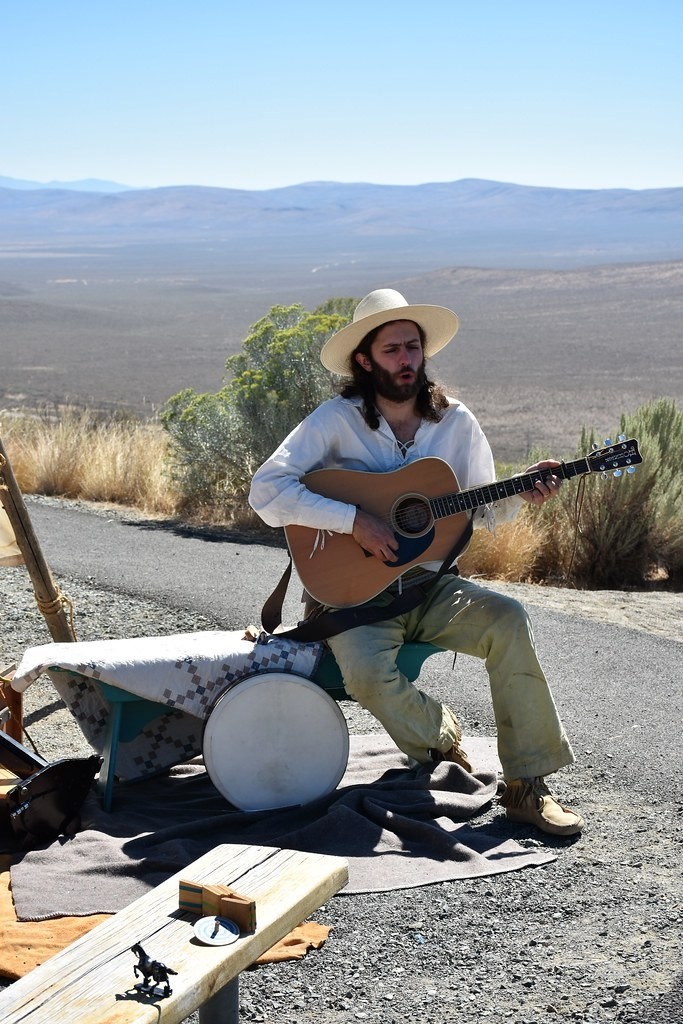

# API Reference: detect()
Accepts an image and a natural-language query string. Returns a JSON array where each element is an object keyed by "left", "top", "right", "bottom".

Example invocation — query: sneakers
[
  {"left": 427, "top": 707, "right": 470, "bottom": 773},
  {"left": 498, "top": 775, "right": 584, "bottom": 836}
]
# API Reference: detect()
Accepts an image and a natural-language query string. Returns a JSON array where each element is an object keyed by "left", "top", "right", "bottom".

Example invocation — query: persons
[{"left": 249, "top": 289, "right": 585, "bottom": 840}]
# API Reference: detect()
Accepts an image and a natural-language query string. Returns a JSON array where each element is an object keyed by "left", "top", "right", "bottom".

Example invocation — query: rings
[{"left": 544, "top": 493, "right": 551, "bottom": 497}]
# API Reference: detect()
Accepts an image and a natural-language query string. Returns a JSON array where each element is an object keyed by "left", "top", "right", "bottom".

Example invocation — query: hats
[{"left": 320, "top": 289, "right": 459, "bottom": 377}]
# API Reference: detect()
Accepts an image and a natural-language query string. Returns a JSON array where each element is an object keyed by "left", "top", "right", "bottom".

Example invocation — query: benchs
[
  {"left": 0, "top": 843, "right": 350, "bottom": 1024},
  {"left": 11, "top": 627, "right": 449, "bottom": 813}
]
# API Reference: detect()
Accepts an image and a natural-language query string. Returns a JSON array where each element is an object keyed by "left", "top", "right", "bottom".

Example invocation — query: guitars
[{"left": 284, "top": 434, "right": 644, "bottom": 610}]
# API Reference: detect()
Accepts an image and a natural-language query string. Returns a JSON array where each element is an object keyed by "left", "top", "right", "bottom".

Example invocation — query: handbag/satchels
[{"left": 0, "top": 756, "right": 104, "bottom": 853}]
